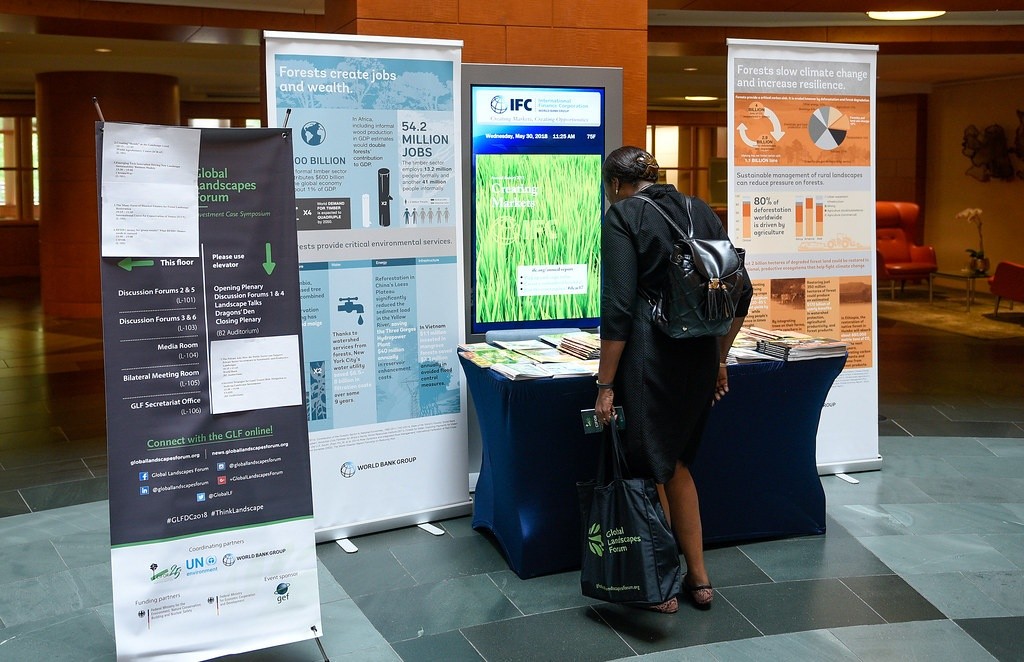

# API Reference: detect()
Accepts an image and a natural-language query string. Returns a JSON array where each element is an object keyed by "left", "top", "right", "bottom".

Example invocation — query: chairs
[
  {"left": 876, "top": 200, "right": 938, "bottom": 300},
  {"left": 486, "top": 328, "right": 581, "bottom": 344},
  {"left": 988, "top": 260, "right": 1024, "bottom": 318}
]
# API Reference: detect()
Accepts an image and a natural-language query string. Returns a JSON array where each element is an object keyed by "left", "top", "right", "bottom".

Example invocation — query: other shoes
[
  {"left": 649, "top": 596, "right": 678, "bottom": 614},
  {"left": 682, "top": 573, "right": 714, "bottom": 610}
]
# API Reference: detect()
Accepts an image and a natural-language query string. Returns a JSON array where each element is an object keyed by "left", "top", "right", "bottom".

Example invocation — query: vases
[{"left": 969, "top": 256, "right": 990, "bottom": 273}]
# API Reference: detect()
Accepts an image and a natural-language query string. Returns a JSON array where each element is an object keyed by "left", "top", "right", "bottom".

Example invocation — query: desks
[
  {"left": 456, "top": 338, "right": 847, "bottom": 580},
  {"left": 928, "top": 270, "right": 1013, "bottom": 312}
]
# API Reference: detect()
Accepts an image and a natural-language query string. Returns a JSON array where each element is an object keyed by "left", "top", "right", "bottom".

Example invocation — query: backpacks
[{"left": 627, "top": 195, "right": 745, "bottom": 337}]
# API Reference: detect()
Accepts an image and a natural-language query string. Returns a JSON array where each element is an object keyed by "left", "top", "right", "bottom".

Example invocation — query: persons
[{"left": 595, "top": 145, "right": 753, "bottom": 614}]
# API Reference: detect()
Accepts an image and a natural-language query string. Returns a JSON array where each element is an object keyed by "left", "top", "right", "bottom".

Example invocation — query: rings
[
  {"left": 719, "top": 387, "right": 723, "bottom": 390},
  {"left": 603, "top": 418, "right": 607, "bottom": 420},
  {"left": 715, "top": 389, "right": 718, "bottom": 392}
]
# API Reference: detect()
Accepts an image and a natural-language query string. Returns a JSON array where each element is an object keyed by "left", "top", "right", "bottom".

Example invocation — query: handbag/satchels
[{"left": 576, "top": 416, "right": 682, "bottom": 604}]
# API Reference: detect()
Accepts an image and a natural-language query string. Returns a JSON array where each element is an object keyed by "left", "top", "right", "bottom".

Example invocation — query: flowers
[{"left": 952, "top": 207, "right": 985, "bottom": 259}]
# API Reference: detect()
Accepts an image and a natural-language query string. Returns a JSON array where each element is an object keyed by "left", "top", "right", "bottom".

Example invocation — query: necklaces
[{"left": 635, "top": 182, "right": 654, "bottom": 194}]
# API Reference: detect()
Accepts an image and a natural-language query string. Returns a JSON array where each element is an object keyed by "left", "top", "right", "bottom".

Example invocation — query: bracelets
[
  {"left": 719, "top": 363, "right": 726, "bottom": 367},
  {"left": 596, "top": 380, "right": 615, "bottom": 389}
]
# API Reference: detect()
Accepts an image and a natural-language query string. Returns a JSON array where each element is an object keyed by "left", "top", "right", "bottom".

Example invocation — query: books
[
  {"left": 458, "top": 332, "right": 602, "bottom": 381},
  {"left": 726, "top": 327, "right": 847, "bottom": 366}
]
